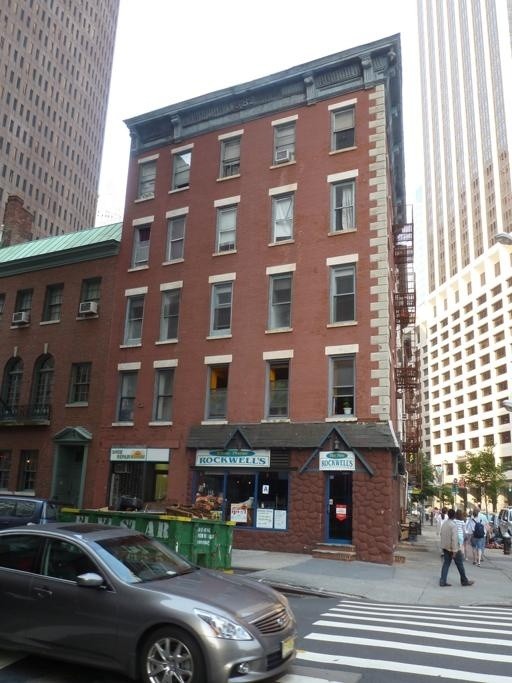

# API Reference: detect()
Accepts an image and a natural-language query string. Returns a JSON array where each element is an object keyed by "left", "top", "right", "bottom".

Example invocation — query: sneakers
[
  {"left": 438, "top": 581, "right": 452, "bottom": 587},
  {"left": 463, "top": 555, "right": 485, "bottom": 567},
  {"left": 462, "top": 580, "right": 474, "bottom": 586}
]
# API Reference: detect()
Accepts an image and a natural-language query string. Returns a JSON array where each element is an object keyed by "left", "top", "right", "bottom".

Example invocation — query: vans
[{"left": 0, "top": 495, "right": 57, "bottom": 530}]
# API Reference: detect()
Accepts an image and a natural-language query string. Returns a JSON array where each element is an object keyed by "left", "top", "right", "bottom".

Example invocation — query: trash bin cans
[{"left": 61, "top": 507, "right": 236, "bottom": 574}]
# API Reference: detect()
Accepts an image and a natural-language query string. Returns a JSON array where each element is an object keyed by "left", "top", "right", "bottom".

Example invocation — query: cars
[
  {"left": 483, "top": 506, "right": 512, "bottom": 540},
  {"left": 0, "top": 522, "right": 298, "bottom": 683}
]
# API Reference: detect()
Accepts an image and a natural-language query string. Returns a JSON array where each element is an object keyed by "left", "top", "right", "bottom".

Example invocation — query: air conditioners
[
  {"left": 78, "top": 301, "right": 97, "bottom": 314},
  {"left": 11, "top": 311, "right": 29, "bottom": 323},
  {"left": 273, "top": 147, "right": 290, "bottom": 163}
]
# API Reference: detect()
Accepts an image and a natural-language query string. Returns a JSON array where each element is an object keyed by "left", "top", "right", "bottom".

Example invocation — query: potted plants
[{"left": 343, "top": 401, "right": 352, "bottom": 414}]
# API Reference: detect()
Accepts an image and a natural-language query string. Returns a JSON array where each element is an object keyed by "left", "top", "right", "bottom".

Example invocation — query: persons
[{"left": 423, "top": 504, "right": 501, "bottom": 585}]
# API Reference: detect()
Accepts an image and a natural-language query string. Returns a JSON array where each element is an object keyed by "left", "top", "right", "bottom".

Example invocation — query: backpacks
[{"left": 470, "top": 518, "right": 484, "bottom": 538}]
